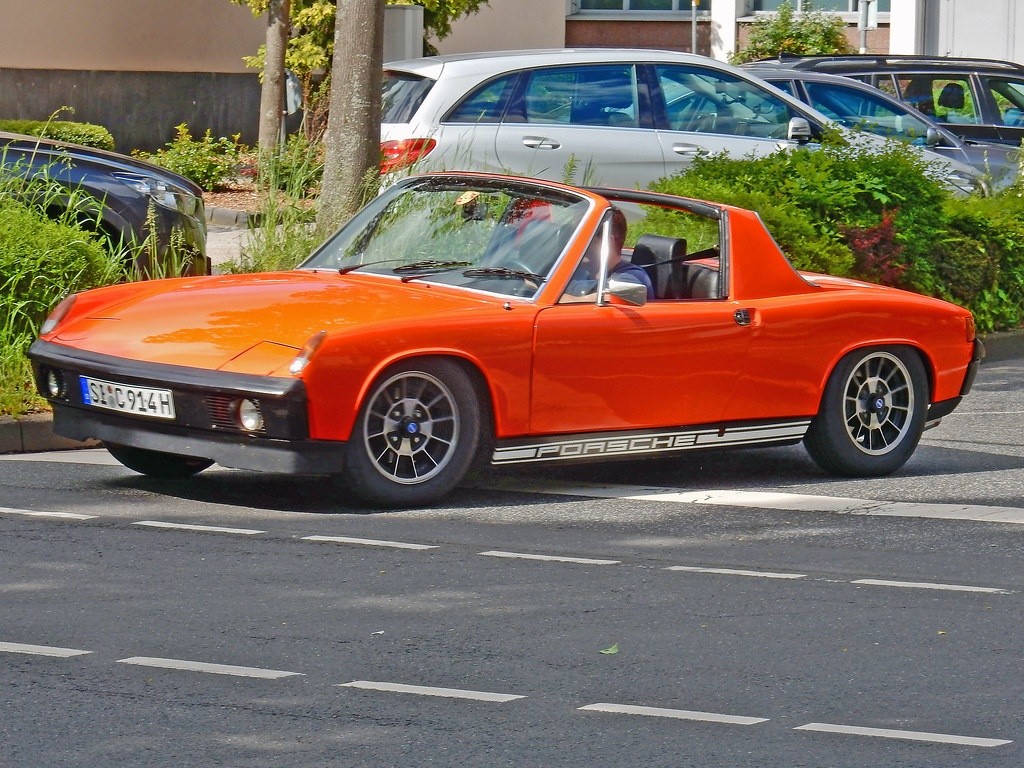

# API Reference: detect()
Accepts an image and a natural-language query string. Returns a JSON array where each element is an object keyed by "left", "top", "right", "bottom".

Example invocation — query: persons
[{"left": 557, "top": 206, "right": 656, "bottom": 304}]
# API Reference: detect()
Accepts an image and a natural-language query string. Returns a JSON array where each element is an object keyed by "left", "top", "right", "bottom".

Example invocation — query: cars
[
  {"left": 537, "top": 68, "right": 1024, "bottom": 191},
  {"left": 1, "top": 136, "right": 213, "bottom": 277}
]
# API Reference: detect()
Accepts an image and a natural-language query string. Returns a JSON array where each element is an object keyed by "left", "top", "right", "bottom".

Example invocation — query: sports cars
[{"left": 24, "top": 171, "right": 986, "bottom": 504}]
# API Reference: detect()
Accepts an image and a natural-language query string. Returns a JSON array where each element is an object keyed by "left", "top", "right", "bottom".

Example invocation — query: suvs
[
  {"left": 370, "top": 46, "right": 990, "bottom": 204},
  {"left": 739, "top": 54, "right": 1024, "bottom": 145}
]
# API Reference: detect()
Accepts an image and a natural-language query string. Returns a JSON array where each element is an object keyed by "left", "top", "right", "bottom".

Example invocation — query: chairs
[
  {"left": 575, "top": 74, "right": 635, "bottom": 125},
  {"left": 519, "top": 222, "right": 573, "bottom": 278},
  {"left": 934, "top": 83, "right": 964, "bottom": 126},
  {"left": 905, "top": 79, "right": 932, "bottom": 117},
  {"left": 631, "top": 234, "right": 687, "bottom": 299}
]
